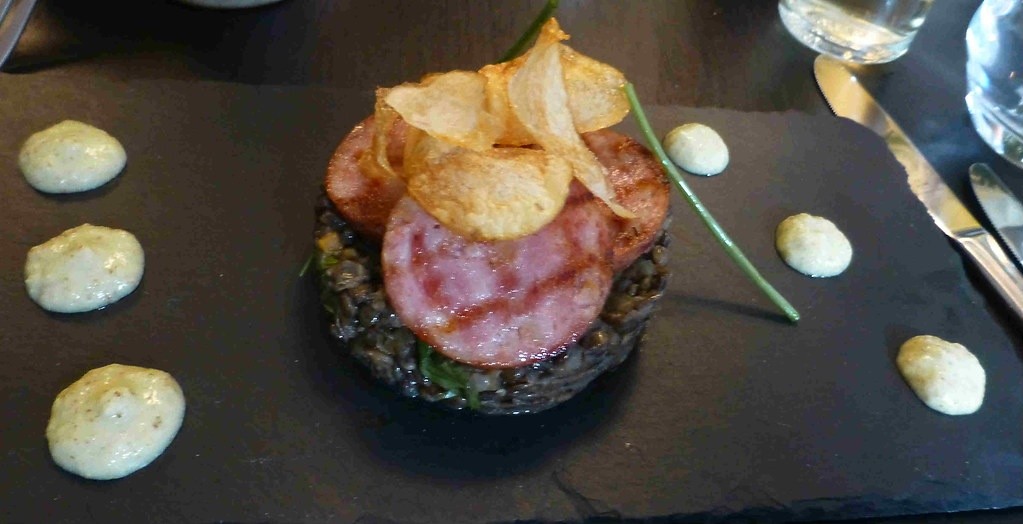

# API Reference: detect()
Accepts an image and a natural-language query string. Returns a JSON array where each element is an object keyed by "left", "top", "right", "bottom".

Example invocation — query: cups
[
  {"left": 965, "top": 0, "right": 1023, "bottom": 169},
  {"left": 777, "top": 1, "right": 935, "bottom": 66}
]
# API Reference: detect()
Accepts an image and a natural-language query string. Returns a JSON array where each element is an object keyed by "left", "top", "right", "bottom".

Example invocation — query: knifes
[
  {"left": 811, "top": 53, "right": 1023, "bottom": 330},
  {"left": 969, "top": 159, "right": 1023, "bottom": 270}
]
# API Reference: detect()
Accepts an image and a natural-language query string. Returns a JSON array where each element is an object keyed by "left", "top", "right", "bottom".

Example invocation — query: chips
[{"left": 369, "top": 17, "right": 631, "bottom": 240}]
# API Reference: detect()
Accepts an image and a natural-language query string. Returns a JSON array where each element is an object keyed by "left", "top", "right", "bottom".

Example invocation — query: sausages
[{"left": 325, "top": 112, "right": 670, "bottom": 367}]
